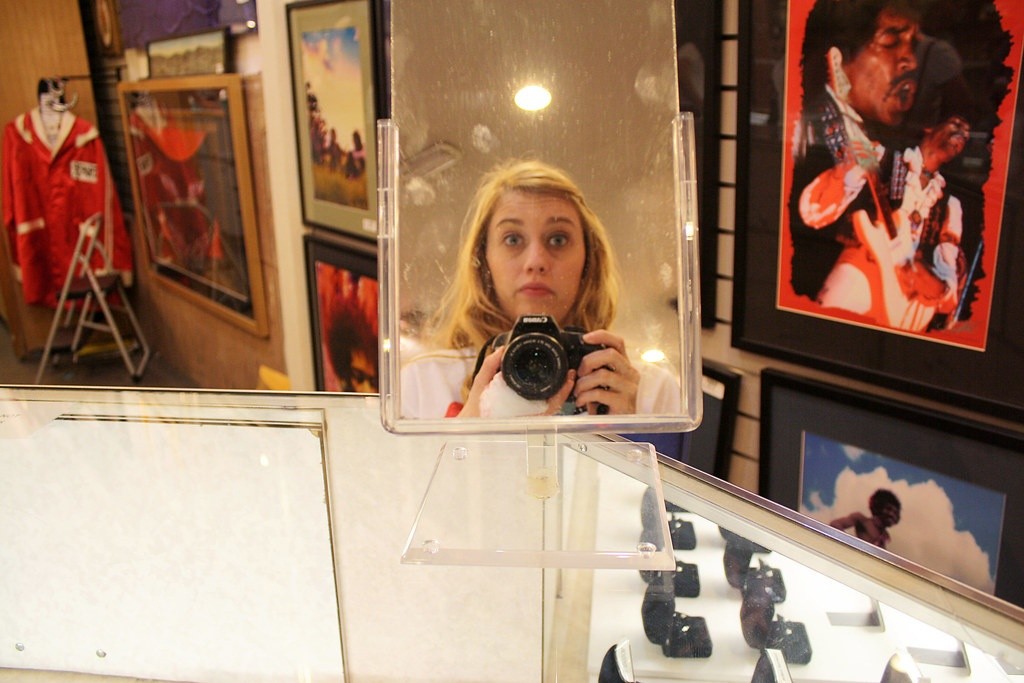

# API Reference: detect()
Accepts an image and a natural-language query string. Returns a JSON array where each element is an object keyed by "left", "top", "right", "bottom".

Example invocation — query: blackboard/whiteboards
[{"left": 1, "top": 397, "right": 351, "bottom": 683}]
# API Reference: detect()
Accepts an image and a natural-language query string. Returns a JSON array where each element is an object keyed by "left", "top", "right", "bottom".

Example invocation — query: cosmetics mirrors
[{"left": 379, "top": 0, "right": 705, "bottom": 434}]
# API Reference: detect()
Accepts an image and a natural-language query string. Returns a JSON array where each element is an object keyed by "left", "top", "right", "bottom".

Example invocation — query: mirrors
[{"left": 1, "top": 1, "right": 388, "bottom": 396}]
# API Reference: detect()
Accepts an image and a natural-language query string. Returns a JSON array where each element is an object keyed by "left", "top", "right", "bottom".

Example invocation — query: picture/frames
[
  {"left": 118, "top": 74, "right": 269, "bottom": 339},
  {"left": 142, "top": 25, "right": 233, "bottom": 80},
  {"left": 286, "top": 0, "right": 392, "bottom": 247},
  {"left": 729, "top": 1, "right": 1024, "bottom": 424},
  {"left": 304, "top": 235, "right": 377, "bottom": 392},
  {"left": 672, "top": 0, "right": 723, "bottom": 328},
  {"left": 618, "top": 354, "right": 742, "bottom": 482},
  {"left": 757, "top": 367, "right": 1023, "bottom": 607}
]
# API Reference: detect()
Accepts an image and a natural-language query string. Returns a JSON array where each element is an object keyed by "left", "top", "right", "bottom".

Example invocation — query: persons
[
  {"left": 401, "top": 159, "right": 680, "bottom": 421},
  {"left": 789, "top": 0, "right": 970, "bottom": 334},
  {"left": 306, "top": 82, "right": 367, "bottom": 181},
  {"left": 325, "top": 304, "right": 378, "bottom": 393},
  {"left": 830, "top": 489, "right": 901, "bottom": 550}
]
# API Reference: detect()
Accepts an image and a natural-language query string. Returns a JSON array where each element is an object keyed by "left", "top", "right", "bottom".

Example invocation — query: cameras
[{"left": 493, "top": 312, "right": 603, "bottom": 400}]
